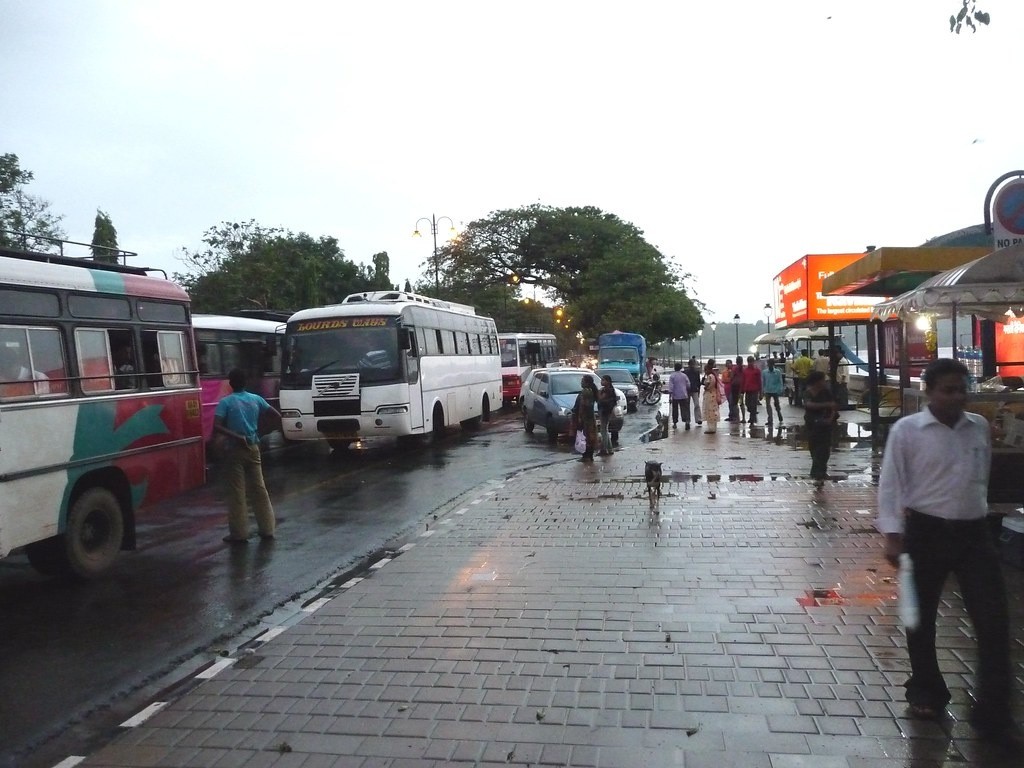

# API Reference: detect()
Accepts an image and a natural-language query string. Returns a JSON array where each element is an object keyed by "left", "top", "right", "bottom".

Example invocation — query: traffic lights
[{"left": 553, "top": 308, "right": 563, "bottom": 324}]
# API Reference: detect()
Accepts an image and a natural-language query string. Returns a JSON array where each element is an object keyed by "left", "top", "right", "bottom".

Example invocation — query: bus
[
  {"left": 0, "top": 228, "right": 204, "bottom": 576},
  {"left": 497, "top": 333, "right": 557, "bottom": 403},
  {"left": 190, "top": 314, "right": 288, "bottom": 459},
  {"left": 266, "top": 291, "right": 504, "bottom": 451}
]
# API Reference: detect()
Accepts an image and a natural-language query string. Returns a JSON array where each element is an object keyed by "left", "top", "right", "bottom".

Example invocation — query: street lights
[
  {"left": 412, "top": 214, "right": 457, "bottom": 297},
  {"left": 504, "top": 276, "right": 518, "bottom": 326},
  {"left": 698, "top": 327, "right": 703, "bottom": 369},
  {"left": 650, "top": 336, "right": 683, "bottom": 367},
  {"left": 710, "top": 322, "right": 717, "bottom": 360},
  {"left": 763, "top": 303, "right": 773, "bottom": 358},
  {"left": 733, "top": 314, "right": 741, "bottom": 356}
]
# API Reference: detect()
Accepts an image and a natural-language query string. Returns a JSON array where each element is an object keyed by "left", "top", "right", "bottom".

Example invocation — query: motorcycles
[{"left": 639, "top": 368, "right": 666, "bottom": 405}]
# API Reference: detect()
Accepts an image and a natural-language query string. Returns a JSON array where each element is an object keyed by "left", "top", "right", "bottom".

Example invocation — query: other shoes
[
  {"left": 724, "top": 417, "right": 732, "bottom": 421},
  {"left": 748, "top": 419, "right": 756, "bottom": 423},
  {"left": 698, "top": 421, "right": 702, "bottom": 424},
  {"left": 222, "top": 534, "right": 245, "bottom": 542},
  {"left": 779, "top": 414, "right": 783, "bottom": 422},
  {"left": 705, "top": 430, "right": 716, "bottom": 434},
  {"left": 749, "top": 424, "right": 754, "bottom": 428},
  {"left": 765, "top": 422, "right": 773, "bottom": 426},
  {"left": 595, "top": 449, "right": 614, "bottom": 456},
  {"left": 258, "top": 533, "right": 274, "bottom": 539},
  {"left": 757, "top": 400, "right": 762, "bottom": 405},
  {"left": 912, "top": 699, "right": 940, "bottom": 718},
  {"left": 739, "top": 419, "right": 746, "bottom": 423},
  {"left": 672, "top": 425, "right": 677, "bottom": 428},
  {"left": 577, "top": 457, "right": 594, "bottom": 462},
  {"left": 685, "top": 424, "right": 690, "bottom": 430}
]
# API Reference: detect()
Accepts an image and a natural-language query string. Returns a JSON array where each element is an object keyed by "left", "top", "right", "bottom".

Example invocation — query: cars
[
  {"left": 651, "top": 370, "right": 676, "bottom": 394},
  {"left": 594, "top": 368, "right": 640, "bottom": 411}
]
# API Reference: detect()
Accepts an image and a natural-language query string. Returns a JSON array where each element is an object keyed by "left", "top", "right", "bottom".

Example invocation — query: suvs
[{"left": 519, "top": 367, "right": 628, "bottom": 442}]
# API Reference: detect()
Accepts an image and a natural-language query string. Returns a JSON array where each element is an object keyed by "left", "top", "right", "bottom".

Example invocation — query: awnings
[{"left": 870, "top": 243, "right": 1024, "bottom": 357}]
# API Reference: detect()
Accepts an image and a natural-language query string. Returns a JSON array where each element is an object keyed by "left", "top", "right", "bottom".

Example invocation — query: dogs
[{"left": 644, "top": 460, "right": 664, "bottom": 512}]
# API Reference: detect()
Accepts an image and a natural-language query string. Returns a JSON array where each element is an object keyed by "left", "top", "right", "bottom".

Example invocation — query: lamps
[
  {"left": 809, "top": 321, "right": 818, "bottom": 332},
  {"left": 1012, "top": 304, "right": 1024, "bottom": 318}
]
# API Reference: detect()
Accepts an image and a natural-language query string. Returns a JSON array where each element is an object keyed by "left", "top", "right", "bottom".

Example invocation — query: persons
[
  {"left": 708, "top": 359, "right": 719, "bottom": 373},
  {"left": 0, "top": 346, "right": 51, "bottom": 394},
  {"left": 803, "top": 369, "right": 836, "bottom": 487},
  {"left": 597, "top": 375, "right": 616, "bottom": 457},
  {"left": 667, "top": 362, "right": 692, "bottom": 430},
  {"left": 836, "top": 352, "right": 849, "bottom": 388},
  {"left": 791, "top": 349, "right": 815, "bottom": 407},
  {"left": 214, "top": 368, "right": 284, "bottom": 545},
  {"left": 729, "top": 356, "right": 746, "bottom": 423},
  {"left": 356, "top": 337, "right": 392, "bottom": 370},
  {"left": 772, "top": 351, "right": 786, "bottom": 363},
  {"left": 873, "top": 358, "right": 1024, "bottom": 743},
  {"left": 691, "top": 356, "right": 703, "bottom": 369},
  {"left": 112, "top": 343, "right": 136, "bottom": 389},
  {"left": 684, "top": 358, "right": 703, "bottom": 425},
  {"left": 703, "top": 364, "right": 721, "bottom": 434},
  {"left": 740, "top": 356, "right": 761, "bottom": 423},
  {"left": 722, "top": 360, "right": 735, "bottom": 421},
  {"left": 816, "top": 349, "right": 828, "bottom": 374},
  {"left": 571, "top": 375, "right": 600, "bottom": 462},
  {"left": 761, "top": 359, "right": 783, "bottom": 425}
]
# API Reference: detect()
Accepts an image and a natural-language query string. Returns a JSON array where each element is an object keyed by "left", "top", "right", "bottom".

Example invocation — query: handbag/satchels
[
  {"left": 575, "top": 429, "right": 586, "bottom": 453},
  {"left": 895, "top": 552, "right": 920, "bottom": 629}
]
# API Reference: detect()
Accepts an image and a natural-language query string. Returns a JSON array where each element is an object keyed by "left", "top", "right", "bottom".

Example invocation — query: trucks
[{"left": 593, "top": 333, "right": 646, "bottom": 382}]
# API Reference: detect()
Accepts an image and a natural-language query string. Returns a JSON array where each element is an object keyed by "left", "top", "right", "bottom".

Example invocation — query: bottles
[
  {"left": 957, "top": 344, "right": 984, "bottom": 378},
  {"left": 920, "top": 369, "right": 926, "bottom": 390}
]
